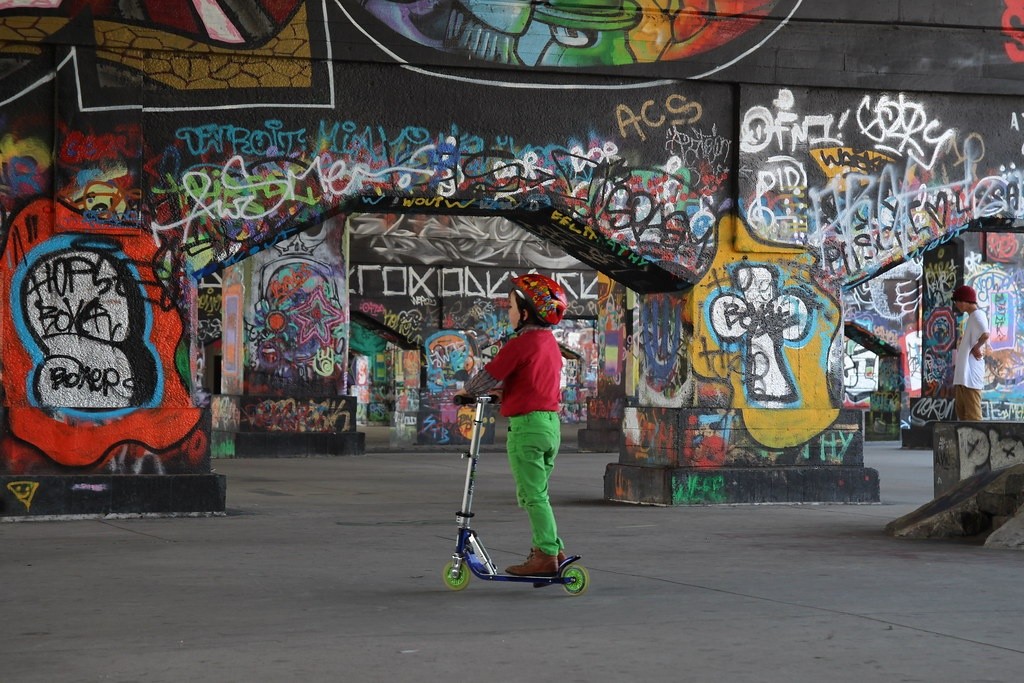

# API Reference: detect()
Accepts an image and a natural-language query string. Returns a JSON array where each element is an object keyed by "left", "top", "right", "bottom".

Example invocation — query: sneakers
[
  {"left": 505, "top": 546, "right": 558, "bottom": 577},
  {"left": 556, "top": 550, "right": 566, "bottom": 565}
]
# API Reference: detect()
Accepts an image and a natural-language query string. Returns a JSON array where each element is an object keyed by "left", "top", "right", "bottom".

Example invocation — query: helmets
[{"left": 512, "top": 274, "right": 567, "bottom": 324}]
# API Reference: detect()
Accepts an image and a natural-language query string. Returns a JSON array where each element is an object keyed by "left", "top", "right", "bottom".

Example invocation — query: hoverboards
[{"left": 442, "top": 393, "right": 590, "bottom": 596}]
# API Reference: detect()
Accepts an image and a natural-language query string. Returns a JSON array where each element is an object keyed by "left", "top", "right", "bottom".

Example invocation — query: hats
[{"left": 953, "top": 286, "right": 976, "bottom": 303}]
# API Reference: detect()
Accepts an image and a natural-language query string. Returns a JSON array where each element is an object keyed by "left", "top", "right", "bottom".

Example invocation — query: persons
[
  {"left": 950, "top": 285, "right": 990, "bottom": 420},
  {"left": 453, "top": 273, "right": 567, "bottom": 574}
]
